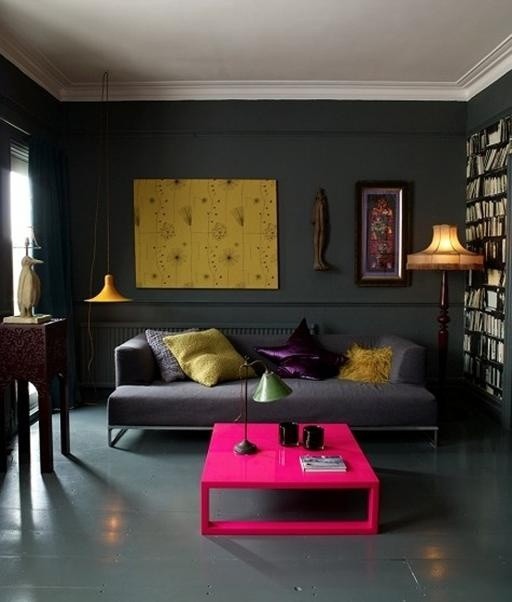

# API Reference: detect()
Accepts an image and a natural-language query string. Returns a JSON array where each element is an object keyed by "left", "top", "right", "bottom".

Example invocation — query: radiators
[{"left": 74, "top": 319, "right": 319, "bottom": 389}]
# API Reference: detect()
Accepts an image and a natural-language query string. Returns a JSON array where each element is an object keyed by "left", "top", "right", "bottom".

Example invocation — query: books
[
  {"left": 299, "top": 455, "right": 347, "bottom": 474},
  {"left": 464, "top": 117, "right": 509, "bottom": 404}
]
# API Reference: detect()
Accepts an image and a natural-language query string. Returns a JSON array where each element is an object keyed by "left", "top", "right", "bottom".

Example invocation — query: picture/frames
[{"left": 353, "top": 177, "right": 416, "bottom": 290}]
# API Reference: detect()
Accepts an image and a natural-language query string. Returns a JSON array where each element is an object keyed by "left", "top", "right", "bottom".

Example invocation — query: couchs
[{"left": 103, "top": 326, "right": 445, "bottom": 453}]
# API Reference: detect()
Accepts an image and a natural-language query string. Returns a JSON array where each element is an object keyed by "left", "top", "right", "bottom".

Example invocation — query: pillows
[
  {"left": 144, "top": 323, "right": 201, "bottom": 384},
  {"left": 339, "top": 342, "right": 392, "bottom": 387},
  {"left": 252, "top": 317, "right": 346, "bottom": 366},
  {"left": 162, "top": 327, "right": 258, "bottom": 389},
  {"left": 270, "top": 356, "right": 343, "bottom": 382}
]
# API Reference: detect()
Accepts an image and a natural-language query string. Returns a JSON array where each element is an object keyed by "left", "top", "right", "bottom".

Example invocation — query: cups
[
  {"left": 303, "top": 425, "right": 324, "bottom": 450},
  {"left": 278, "top": 421, "right": 300, "bottom": 445}
]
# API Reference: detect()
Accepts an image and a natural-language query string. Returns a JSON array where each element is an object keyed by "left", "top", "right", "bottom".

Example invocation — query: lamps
[
  {"left": 83, "top": 66, "right": 131, "bottom": 307},
  {"left": 231, "top": 354, "right": 293, "bottom": 457},
  {"left": 404, "top": 219, "right": 484, "bottom": 439}
]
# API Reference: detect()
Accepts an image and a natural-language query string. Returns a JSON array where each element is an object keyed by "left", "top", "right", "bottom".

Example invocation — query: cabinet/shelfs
[
  {"left": 458, "top": 102, "right": 512, "bottom": 415},
  {"left": 0, "top": 315, "right": 76, "bottom": 481}
]
